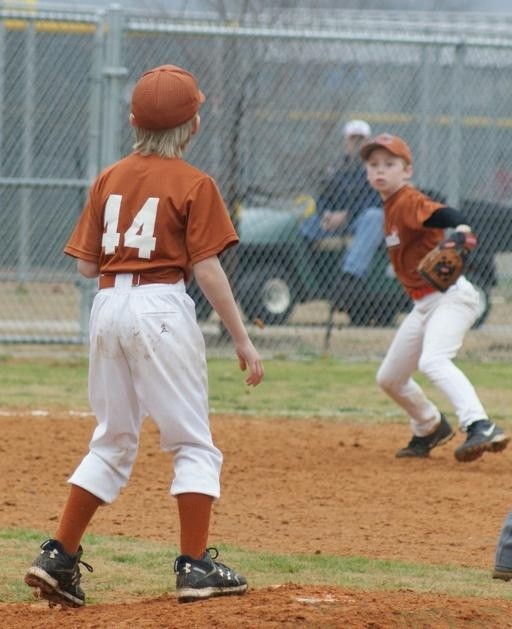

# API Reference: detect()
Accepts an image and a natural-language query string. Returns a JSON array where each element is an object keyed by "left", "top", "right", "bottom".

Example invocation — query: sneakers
[
  {"left": 174, "top": 547, "right": 248, "bottom": 602},
  {"left": 396, "top": 412, "right": 454, "bottom": 458},
  {"left": 455, "top": 419, "right": 511, "bottom": 463},
  {"left": 24, "top": 539, "right": 93, "bottom": 606}
]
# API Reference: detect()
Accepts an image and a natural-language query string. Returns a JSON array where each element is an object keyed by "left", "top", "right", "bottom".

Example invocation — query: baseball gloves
[{"left": 415, "top": 240, "right": 465, "bottom": 292}]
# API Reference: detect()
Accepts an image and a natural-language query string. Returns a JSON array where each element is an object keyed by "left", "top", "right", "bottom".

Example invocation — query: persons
[
  {"left": 359, "top": 133, "right": 512, "bottom": 466},
  {"left": 295, "top": 119, "right": 388, "bottom": 280},
  {"left": 21, "top": 62, "right": 266, "bottom": 611}
]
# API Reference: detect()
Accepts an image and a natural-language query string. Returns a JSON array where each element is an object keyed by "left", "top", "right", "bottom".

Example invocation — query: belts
[
  {"left": 99, "top": 272, "right": 151, "bottom": 288},
  {"left": 409, "top": 288, "right": 433, "bottom": 300}
]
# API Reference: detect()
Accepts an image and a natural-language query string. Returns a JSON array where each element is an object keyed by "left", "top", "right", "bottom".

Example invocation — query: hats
[
  {"left": 345, "top": 119, "right": 371, "bottom": 140},
  {"left": 359, "top": 132, "right": 412, "bottom": 164},
  {"left": 132, "top": 65, "right": 206, "bottom": 131}
]
